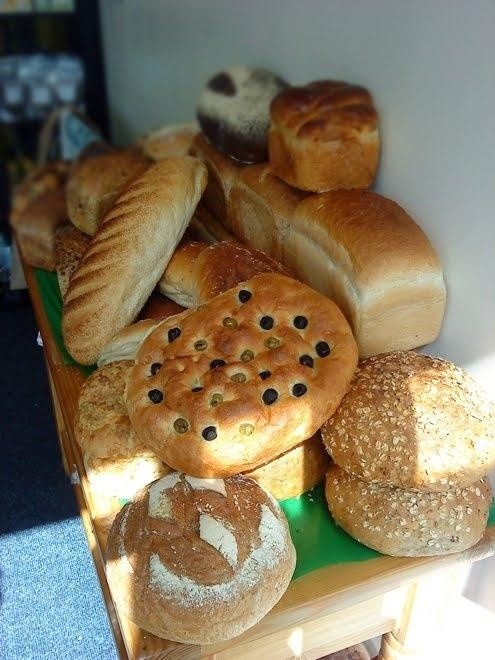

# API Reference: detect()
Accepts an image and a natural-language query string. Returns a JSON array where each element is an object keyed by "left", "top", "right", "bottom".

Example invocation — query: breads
[{"left": 12, "top": 64, "right": 495, "bottom": 645}]
